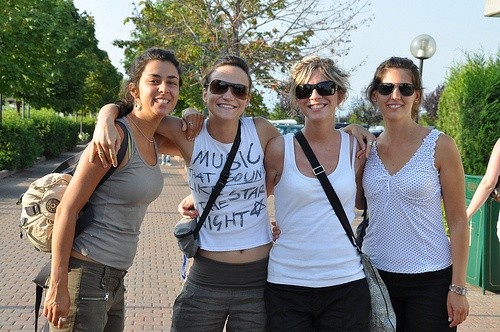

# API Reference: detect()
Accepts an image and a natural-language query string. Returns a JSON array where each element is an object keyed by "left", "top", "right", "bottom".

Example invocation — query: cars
[{"left": 267, "top": 119, "right": 384, "bottom": 138}]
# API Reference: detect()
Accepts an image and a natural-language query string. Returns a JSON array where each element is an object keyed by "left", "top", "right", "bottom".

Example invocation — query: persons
[
  {"left": 270, "top": 58, "right": 469, "bottom": 332},
  {"left": 177, "top": 57, "right": 371, "bottom": 332},
  {"left": 88, "top": 56, "right": 375, "bottom": 332},
  {"left": 34, "top": 48, "right": 205, "bottom": 332},
  {"left": 466, "top": 137, "right": 500, "bottom": 243}
]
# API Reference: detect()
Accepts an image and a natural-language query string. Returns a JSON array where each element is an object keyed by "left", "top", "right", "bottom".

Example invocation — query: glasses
[
  {"left": 376, "top": 83, "right": 415, "bottom": 96},
  {"left": 205, "top": 80, "right": 249, "bottom": 100},
  {"left": 295, "top": 81, "right": 338, "bottom": 99}
]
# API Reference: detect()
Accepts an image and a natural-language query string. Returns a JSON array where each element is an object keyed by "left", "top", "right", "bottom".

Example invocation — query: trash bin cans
[{"left": 464, "top": 175, "right": 500, "bottom": 295}]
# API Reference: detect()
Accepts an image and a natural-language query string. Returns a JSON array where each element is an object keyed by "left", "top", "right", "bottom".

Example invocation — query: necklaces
[{"left": 126, "top": 114, "right": 155, "bottom": 143}]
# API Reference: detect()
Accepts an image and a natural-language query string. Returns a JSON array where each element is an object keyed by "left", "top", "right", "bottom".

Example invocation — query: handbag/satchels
[
  {"left": 15, "top": 172, "right": 92, "bottom": 253},
  {"left": 361, "top": 253, "right": 397, "bottom": 332},
  {"left": 357, "top": 220, "right": 368, "bottom": 249},
  {"left": 174, "top": 218, "right": 198, "bottom": 258}
]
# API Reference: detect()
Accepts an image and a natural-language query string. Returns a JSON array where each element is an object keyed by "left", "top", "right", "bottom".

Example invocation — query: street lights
[{"left": 410, "top": 34, "right": 436, "bottom": 124}]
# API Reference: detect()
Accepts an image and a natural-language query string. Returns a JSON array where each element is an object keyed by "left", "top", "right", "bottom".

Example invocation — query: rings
[
  {"left": 367, "top": 141, "right": 373, "bottom": 146},
  {"left": 58, "top": 317, "right": 66, "bottom": 323},
  {"left": 187, "top": 121, "right": 193, "bottom": 126}
]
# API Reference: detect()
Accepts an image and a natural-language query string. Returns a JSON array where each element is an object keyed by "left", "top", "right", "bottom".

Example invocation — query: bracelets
[{"left": 448, "top": 283, "right": 468, "bottom": 296}]
